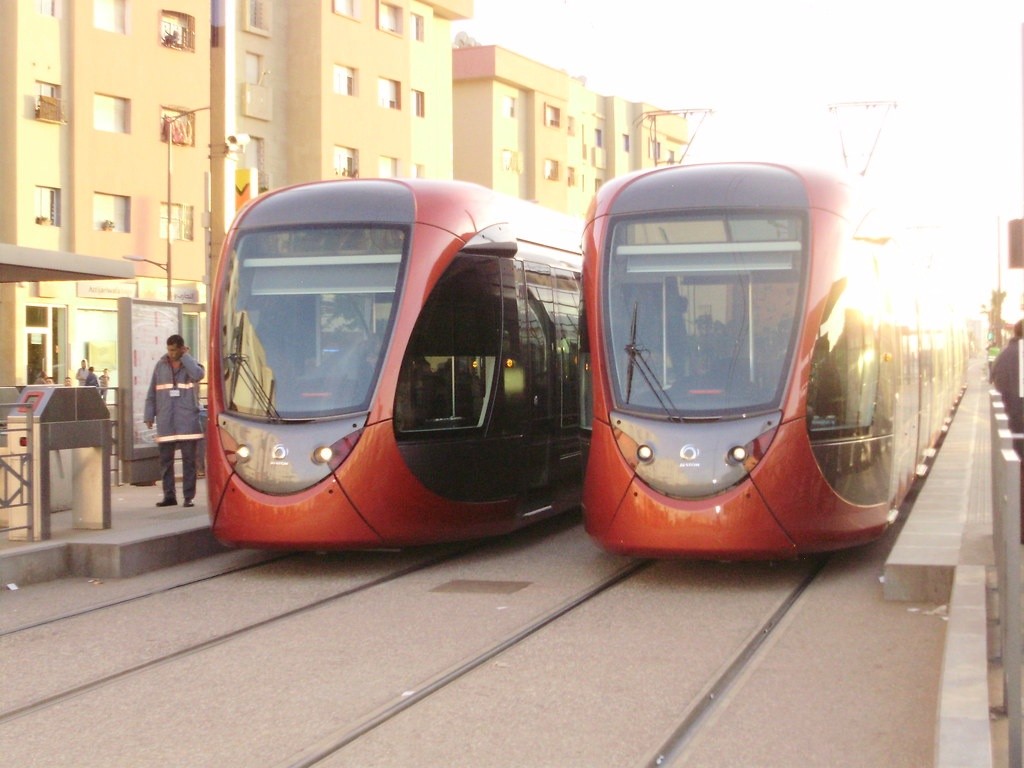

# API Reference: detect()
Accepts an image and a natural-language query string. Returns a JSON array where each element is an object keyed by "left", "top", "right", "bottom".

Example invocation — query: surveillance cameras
[{"left": 228, "top": 133, "right": 251, "bottom": 145}]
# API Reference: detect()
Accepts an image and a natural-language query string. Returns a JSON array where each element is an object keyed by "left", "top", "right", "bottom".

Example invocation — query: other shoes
[
  {"left": 156, "top": 499, "right": 177, "bottom": 507},
  {"left": 184, "top": 500, "right": 194, "bottom": 507}
]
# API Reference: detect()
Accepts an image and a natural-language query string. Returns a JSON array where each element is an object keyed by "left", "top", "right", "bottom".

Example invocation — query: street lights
[{"left": 118, "top": 103, "right": 212, "bottom": 302}]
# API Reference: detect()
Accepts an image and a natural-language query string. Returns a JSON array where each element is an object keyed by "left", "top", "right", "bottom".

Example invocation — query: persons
[
  {"left": 334, "top": 332, "right": 384, "bottom": 409},
  {"left": 99, "top": 369, "right": 110, "bottom": 399},
  {"left": 29, "top": 344, "right": 52, "bottom": 384},
  {"left": 144, "top": 335, "right": 205, "bottom": 506},
  {"left": 76, "top": 360, "right": 89, "bottom": 386},
  {"left": 986, "top": 320, "right": 1024, "bottom": 545},
  {"left": 86, "top": 367, "right": 99, "bottom": 387},
  {"left": 65, "top": 377, "right": 72, "bottom": 386},
  {"left": 398, "top": 354, "right": 483, "bottom": 427}
]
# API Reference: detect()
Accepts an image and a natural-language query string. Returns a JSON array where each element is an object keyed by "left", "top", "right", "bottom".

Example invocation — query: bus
[
  {"left": 575, "top": 103, "right": 983, "bottom": 559},
  {"left": 197, "top": 108, "right": 733, "bottom": 555}
]
